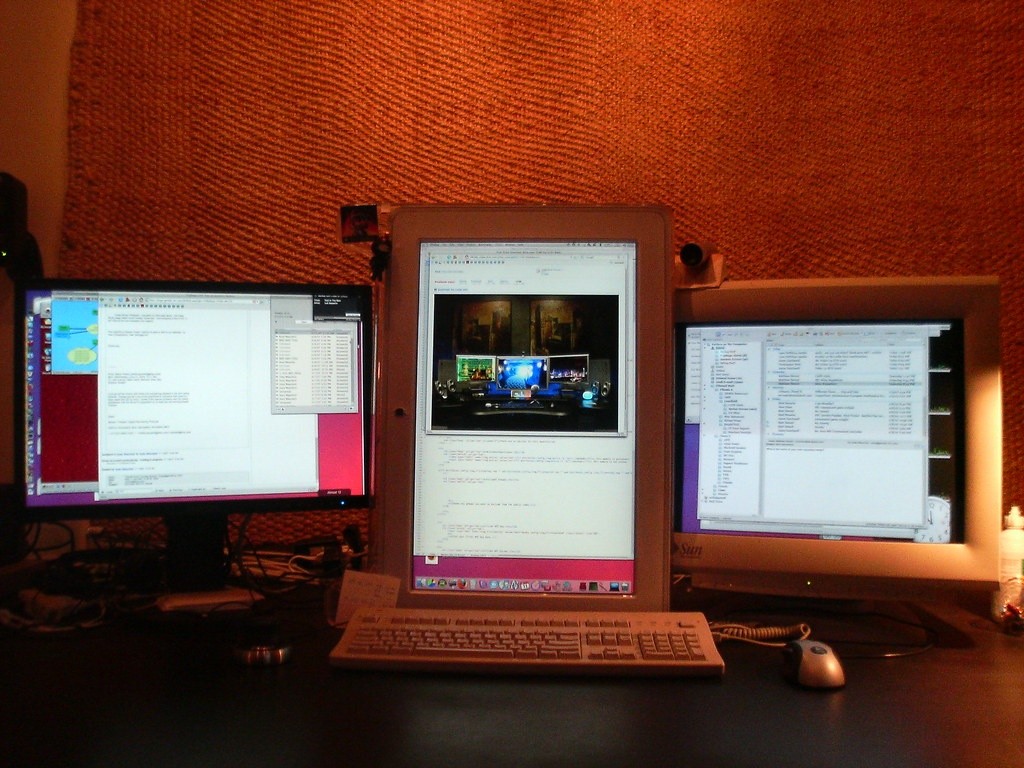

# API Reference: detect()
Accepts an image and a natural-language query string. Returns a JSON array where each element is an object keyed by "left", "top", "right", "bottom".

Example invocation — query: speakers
[
  {"left": 601, "top": 382, "right": 610, "bottom": 400},
  {"left": 434, "top": 379, "right": 456, "bottom": 400},
  {"left": 591, "top": 381, "right": 601, "bottom": 401}
]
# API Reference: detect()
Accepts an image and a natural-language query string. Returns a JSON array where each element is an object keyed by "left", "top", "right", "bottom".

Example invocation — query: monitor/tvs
[
  {"left": 675, "top": 276, "right": 1001, "bottom": 655},
  {"left": 456, "top": 354, "right": 589, "bottom": 400},
  {"left": 381, "top": 203, "right": 673, "bottom": 615},
  {"left": 15, "top": 278, "right": 374, "bottom": 602}
]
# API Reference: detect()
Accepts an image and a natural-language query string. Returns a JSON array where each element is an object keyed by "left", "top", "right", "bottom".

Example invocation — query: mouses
[{"left": 785, "top": 639, "right": 844, "bottom": 688}]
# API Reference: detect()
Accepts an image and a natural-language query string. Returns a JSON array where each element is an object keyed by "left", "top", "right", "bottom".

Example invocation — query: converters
[{"left": 157, "top": 591, "right": 264, "bottom": 612}]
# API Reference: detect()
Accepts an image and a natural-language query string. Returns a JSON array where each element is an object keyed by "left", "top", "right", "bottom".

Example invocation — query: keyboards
[{"left": 328, "top": 605, "right": 724, "bottom": 675}]
